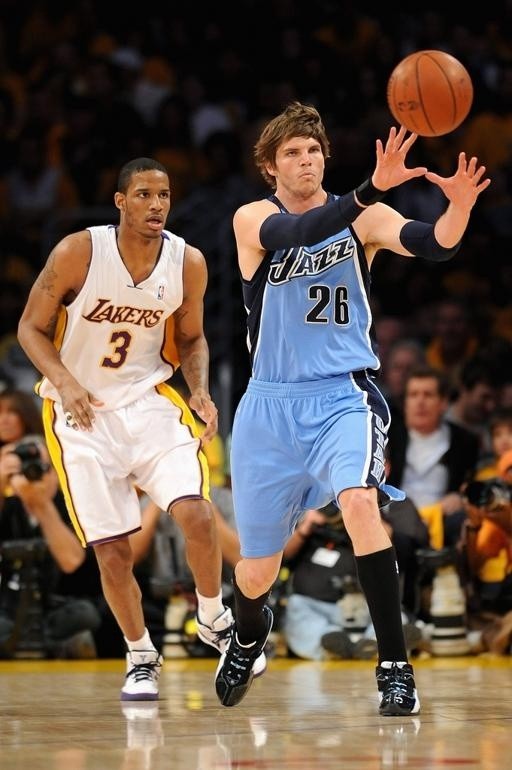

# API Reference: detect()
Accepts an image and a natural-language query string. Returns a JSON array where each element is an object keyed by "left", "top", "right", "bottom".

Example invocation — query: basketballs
[{"left": 387, "top": 51, "right": 472, "bottom": 138}]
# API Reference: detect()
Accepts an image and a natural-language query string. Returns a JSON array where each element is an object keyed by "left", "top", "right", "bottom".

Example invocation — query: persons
[
  {"left": 112, "top": 705, "right": 269, "bottom": 770},
  {"left": 2, "top": 1, "right": 512, "bottom": 657},
  {"left": 212, "top": 702, "right": 421, "bottom": 770},
  {"left": 17, "top": 158, "right": 267, "bottom": 703},
  {"left": 203, "top": 99, "right": 491, "bottom": 720}
]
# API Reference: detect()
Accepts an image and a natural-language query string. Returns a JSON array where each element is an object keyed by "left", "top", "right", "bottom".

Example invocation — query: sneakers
[
  {"left": 117, "top": 702, "right": 164, "bottom": 752},
  {"left": 375, "top": 717, "right": 422, "bottom": 760},
  {"left": 55, "top": 631, "right": 102, "bottom": 661},
  {"left": 316, "top": 632, "right": 381, "bottom": 661},
  {"left": 216, "top": 609, "right": 272, "bottom": 706},
  {"left": 375, "top": 660, "right": 422, "bottom": 717},
  {"left": 120, "top": 646, "right": 166, "bottom": 702},
  {"left": 192, "top": 605, "right": 270, "bottom": 676},
  {"left": 212, "top": 705, "right": 267, "bottom": 770}
]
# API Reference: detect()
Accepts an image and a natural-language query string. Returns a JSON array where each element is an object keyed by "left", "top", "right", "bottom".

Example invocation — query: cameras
[
  {"left": 465, "top": 477, "right": 512, "bottom": 506},
  {"left": 15, "top": 442, "right": 43, "bottom": 481}
]
